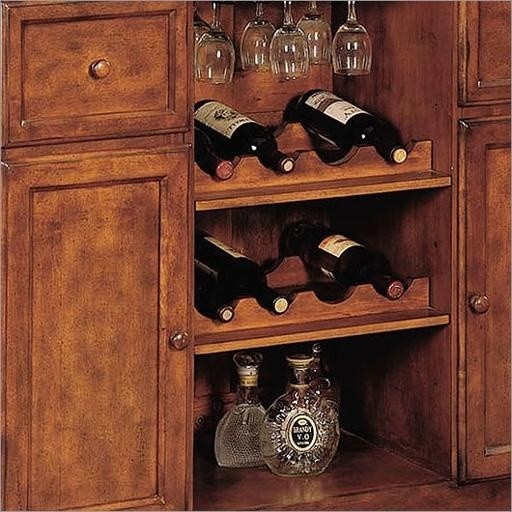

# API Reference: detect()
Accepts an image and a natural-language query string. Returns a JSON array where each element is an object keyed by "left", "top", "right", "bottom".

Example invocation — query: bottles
[
  {"left": 194, "top": 229, "right": 289, "bottom": 323},
  {"left": 297, "top": 88, "right": 408, "bottom": 163},
  {"left": 214, "top": 340, "right": 343, "bottom": 477},
  {"left": 194, "top": 99, "right": 296, "bottom": 181},
  {"left": 286, "top": 221, "right": 405, "bottom": 299}
]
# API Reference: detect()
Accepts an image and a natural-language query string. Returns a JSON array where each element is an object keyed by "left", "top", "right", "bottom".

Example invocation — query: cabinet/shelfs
[{"left": 0, "top": 0, "right": 512, "bottom": 512}]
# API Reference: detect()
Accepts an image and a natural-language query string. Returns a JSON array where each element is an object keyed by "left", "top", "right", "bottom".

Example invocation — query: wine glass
[{"left": 195, "top": 1, "right": 374, "bottom": 85}]
